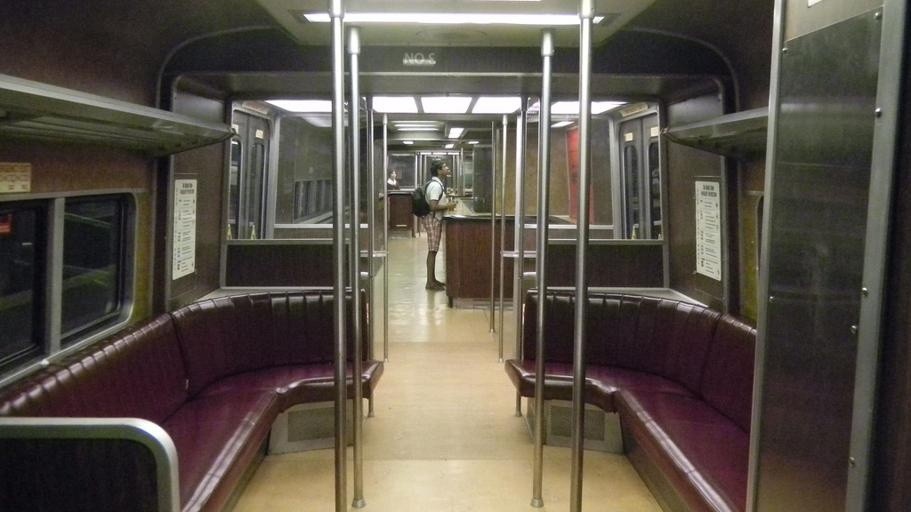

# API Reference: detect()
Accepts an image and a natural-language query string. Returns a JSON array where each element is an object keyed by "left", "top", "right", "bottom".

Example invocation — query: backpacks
[{"left": 409, "top": 179, "right": 443, "bottom": 218}]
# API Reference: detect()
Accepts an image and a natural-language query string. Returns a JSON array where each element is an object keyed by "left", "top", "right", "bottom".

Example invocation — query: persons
[
  {"left": 387, "top": 169, "right": 401, "bottom": 190},
  {"left": 421, "top": 159, "right": 457, "bottom": 292}
]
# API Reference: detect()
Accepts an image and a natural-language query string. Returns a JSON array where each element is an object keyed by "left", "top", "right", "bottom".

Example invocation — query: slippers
[{"left": 425, "top": 280, "right": 447, "bottom": 291}]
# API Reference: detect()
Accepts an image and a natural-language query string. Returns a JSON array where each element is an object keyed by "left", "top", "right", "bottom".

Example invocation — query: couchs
[
  {"left": 1, "top": 287, "right": 384, "bottom": 511},
  {"left": 504, "top": 289, "right": 760, "bottom": 510}
]
